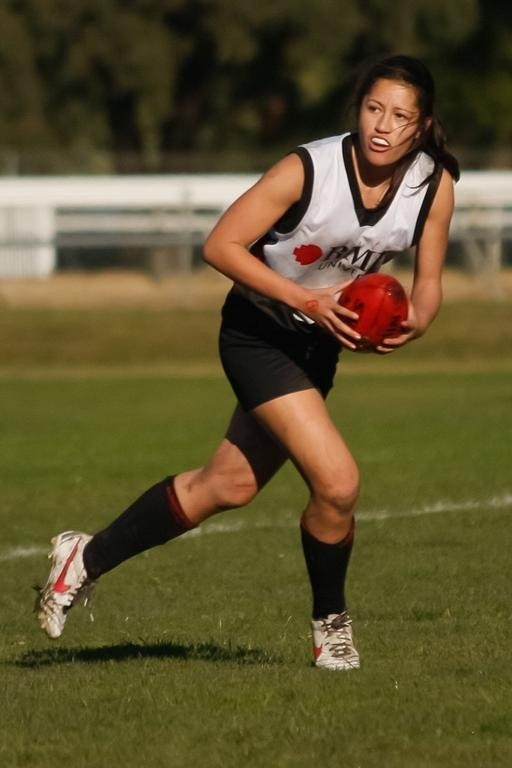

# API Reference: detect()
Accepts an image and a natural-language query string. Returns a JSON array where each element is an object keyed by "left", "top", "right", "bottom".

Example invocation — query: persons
[{"left": 36, "top": 55, "right": 461, "bottom": 670}]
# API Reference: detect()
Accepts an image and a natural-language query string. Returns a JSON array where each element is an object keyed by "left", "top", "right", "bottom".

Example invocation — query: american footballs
[{"left": 334, "top": 274, "right": 408, "bottom": 353}]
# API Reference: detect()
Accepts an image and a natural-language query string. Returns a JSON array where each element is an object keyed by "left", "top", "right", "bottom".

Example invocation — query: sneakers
[
  {"left": 35, "top": 529, "right": 93, "bottom": 639},
  {"left": 308, "top": 611, "right": 360, "bottom": 669}
]
